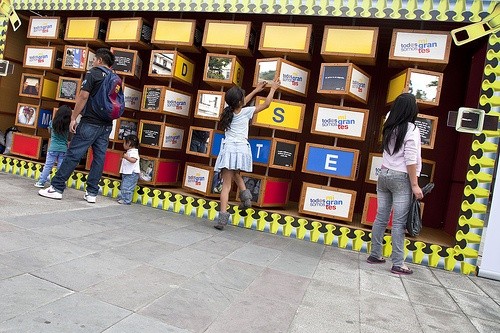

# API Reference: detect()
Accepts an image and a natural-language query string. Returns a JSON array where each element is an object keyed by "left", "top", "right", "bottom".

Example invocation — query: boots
[
  {"left": 214, "top": 212, "right": 230, "bottom": 229},
  {"left": 238, "top": 189, "right": 252, "bottom": 209}
]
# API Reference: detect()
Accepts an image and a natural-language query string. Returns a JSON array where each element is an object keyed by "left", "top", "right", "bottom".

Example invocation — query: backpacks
[{"left": 90, "top": 66, "right": 125, "bottom": 120}]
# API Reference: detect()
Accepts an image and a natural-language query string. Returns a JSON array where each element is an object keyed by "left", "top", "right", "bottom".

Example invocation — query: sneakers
[
  {"left": 34, "top": 182, "right": 44, "bottom": 187},
  {"left": 84, "top": 194, "right": 96, "bottom": 202},
  {"left": 38, "top": 185, "right": 62, "bottom": 199}
]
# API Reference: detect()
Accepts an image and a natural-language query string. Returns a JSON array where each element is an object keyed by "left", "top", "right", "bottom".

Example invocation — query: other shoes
[
  {"left": 117, "top": 199, "right": 128, "bottom": 204},
  {"left": 367, "top": 256, "right": 385, "bottom": 263},
  {"left": 391, "top": 266, "right": 412, "bottom": 273}
]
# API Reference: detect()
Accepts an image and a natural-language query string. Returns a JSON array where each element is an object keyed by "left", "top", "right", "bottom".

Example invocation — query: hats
[{"left": 23, "top": 106, "right": 29, "bottom": 111}]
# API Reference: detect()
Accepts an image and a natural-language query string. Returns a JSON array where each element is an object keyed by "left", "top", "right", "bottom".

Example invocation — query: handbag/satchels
[{"left": 406, "top": 181, "right": 435, "bottom": 236}]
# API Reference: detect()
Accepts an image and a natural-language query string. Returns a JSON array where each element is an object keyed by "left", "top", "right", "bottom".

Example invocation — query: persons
[
  {"left": 214, "top": 78, "right": 281, "bottom": 230},
  {"left": 34, "top": 105, "right": 72, "bottom": 188},
  {"left": 244, "top": 177, "right": 261, "bottom": 203},
  {"left": 367, "top": 93, "right": 424, "bottom": 275},
  {"left": 39, "top": 49, "right": 115, "bottom": 203},
  {"left": 117, "top": 134, "right": 140, "bottom": 205}
]
